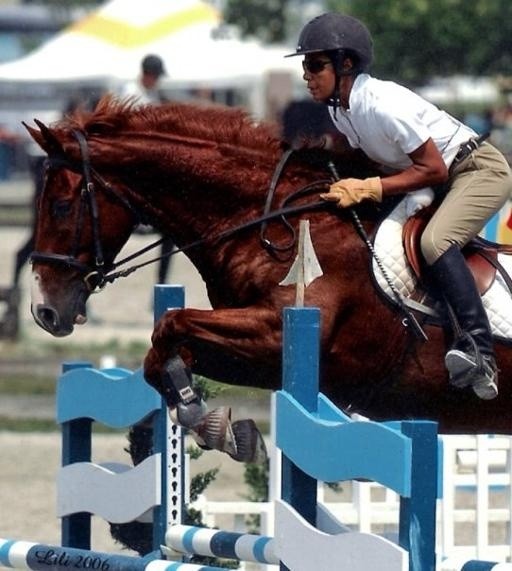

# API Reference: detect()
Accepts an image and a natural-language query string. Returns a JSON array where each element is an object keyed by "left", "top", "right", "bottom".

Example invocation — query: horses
[{"left": 19, "top": 92, "right": 512, "bottom": 467}]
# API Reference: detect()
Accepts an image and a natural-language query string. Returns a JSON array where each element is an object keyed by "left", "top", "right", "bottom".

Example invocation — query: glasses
[{"left": 301, "top": 59, "right": 332, "bottom": 73}]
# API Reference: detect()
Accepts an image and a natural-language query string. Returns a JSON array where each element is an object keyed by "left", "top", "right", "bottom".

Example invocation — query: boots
[{"left": 432, "top": 244, "right": 498, "bottom": 401}]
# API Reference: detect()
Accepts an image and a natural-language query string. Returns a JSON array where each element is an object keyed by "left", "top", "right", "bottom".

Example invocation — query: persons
[
  {"left": 280, "top": 8, "right": 511, "bottom": 403},
  {"left": 118, "top": 52, "right": 167, "bottom": 236}
]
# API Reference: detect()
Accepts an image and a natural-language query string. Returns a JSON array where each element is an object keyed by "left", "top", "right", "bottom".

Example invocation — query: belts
[{"left": 448, "top": 128, "right": 493, "bottom": 178}]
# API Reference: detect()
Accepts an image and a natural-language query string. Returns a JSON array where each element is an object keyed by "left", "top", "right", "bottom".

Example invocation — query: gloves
[{"left": 318, "top": 175, "right": 385, "bottom": 208}]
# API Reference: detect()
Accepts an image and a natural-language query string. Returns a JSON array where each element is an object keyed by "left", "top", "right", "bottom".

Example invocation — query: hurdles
[{"left": 0, "top": 284, "right": 511, "bottom": 571}]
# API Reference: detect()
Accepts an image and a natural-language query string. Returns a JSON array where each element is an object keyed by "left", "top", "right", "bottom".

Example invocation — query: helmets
[
  {"left": 141, "top": 54, "right": 166, "bottom": 77},
  {"left": 284, "top": 12, "right": 374, "bottom": 65}
]
